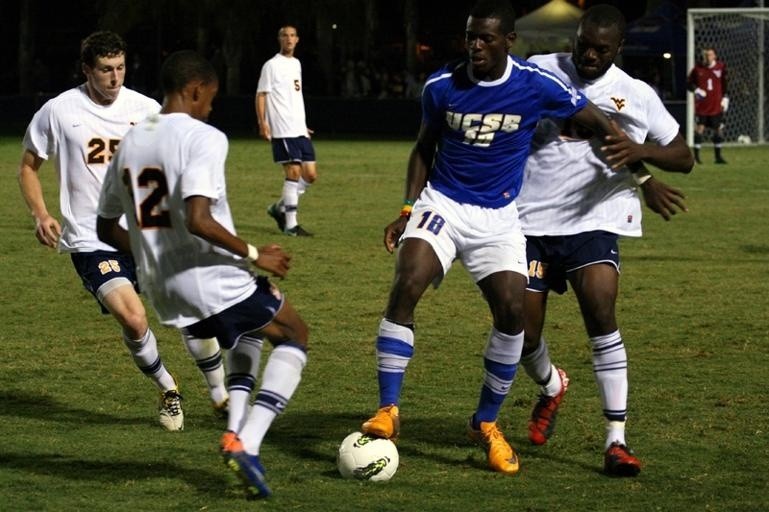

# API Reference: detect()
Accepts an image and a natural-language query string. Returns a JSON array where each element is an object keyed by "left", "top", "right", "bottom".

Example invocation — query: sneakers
[
  {"left": 219, "top": 430, "right": 272, "bottom": 499},
  {"left": 268, "top": 205, "right": 285, "bottom": 232},
  {"left": 285, "top": 225, "right": 313, "bottom": 238},
  {"left": 210, "top": 397, "right": 229, "bottom": 420},
  {"left": 466, "top": 413, "right": 519, "bottom": 475},
  {"left": 361, "top": 404, "right": 400, "bottom": 444},
  {"left": 159, "top": 373, "right": 184, "bottom": 431},
  {"left": 529, "top": 366, "right": 569, "bottom": 445},
  {"left": 604, "top": 441, "right": 641, "bottom": 478}
]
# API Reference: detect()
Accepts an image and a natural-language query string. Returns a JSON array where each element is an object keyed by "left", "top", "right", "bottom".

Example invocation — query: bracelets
[
  {"left": 400, "top": 199, "right": 415, "bottom": 218},
  {"left": 242, "top": 242, "right": 259, "bottom": 263},
  {"left": 632, "top": 168, "right": 651, "bottom": 188}
]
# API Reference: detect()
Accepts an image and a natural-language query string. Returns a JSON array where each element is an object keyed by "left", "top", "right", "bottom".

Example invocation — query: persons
[
  {"left": 254, "top": 25, "right": 316, "bottom": 237},
  {"left": 648, "top": 67, "right": 674, "bottom": 102},
  {"left": 96, "top": 50, "right": 309, "bottom": 501},
  {"left": 360, "top": 5, "right": 690, "bottom": 476},
  {"left": 682, "top": 44, "right": 729, "bottom": 166},
  {"left": 513, "top": 7, "right": 696, "bottom": 479},
  {"left": 341, "top": 52, "right": 429, "bottom": 102},
  {"left": 16, "top": 30, "right": 229, "bottom": 433}
]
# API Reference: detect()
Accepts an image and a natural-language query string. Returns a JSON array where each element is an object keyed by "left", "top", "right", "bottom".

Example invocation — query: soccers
[
  {"left": 738, "top": 134, "right": 752, "bottom": 144},
  {"left": 336, "top": 432, "right": 399, "bottom": 483}
]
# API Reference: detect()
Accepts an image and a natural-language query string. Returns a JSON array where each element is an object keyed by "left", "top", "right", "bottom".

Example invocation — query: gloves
[
  {"left": 720, "top": 97, "right": 730, "bottom": 113},
  {"left": 693, "top": 87, "right": 706, "bottom": 100}
]
